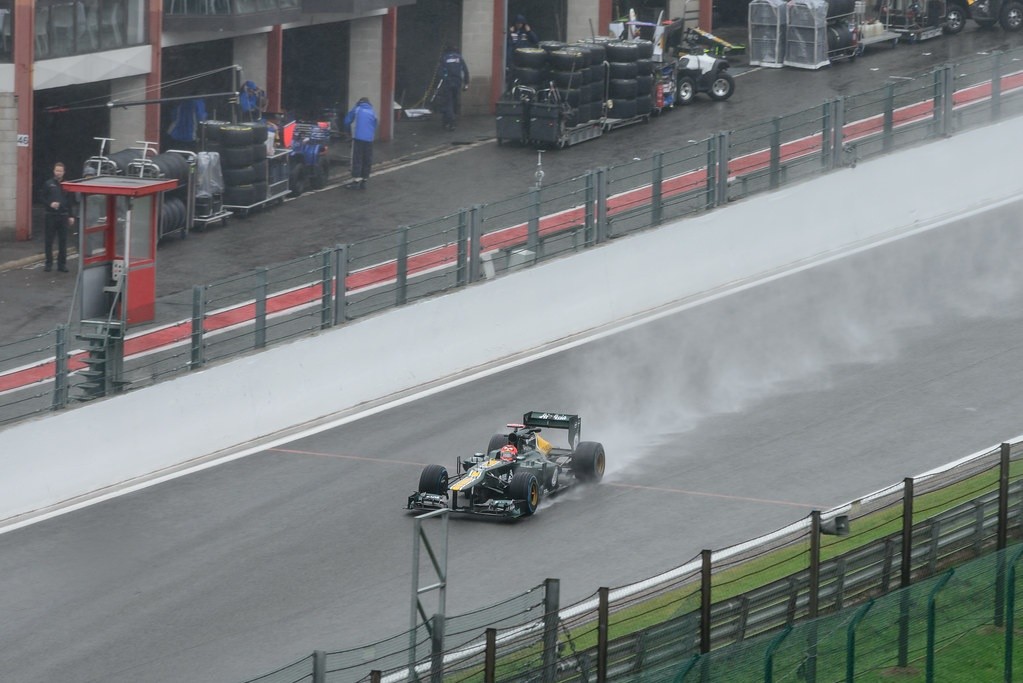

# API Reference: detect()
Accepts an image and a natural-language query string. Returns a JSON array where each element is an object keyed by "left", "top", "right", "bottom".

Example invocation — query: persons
[
  {"left": 344, "top": 97, "right": 378, "bottom": 190},
  {"left": 39, "top": 162, "right": 77, "bottom": 272},
  {"left": 500, "top": 444, "right": 518, "bottom": 462},
  {"left": 440, "top": 47, "right": 469, "bottom": 131},
  {"left": 170, "top": 89, "right": 207, "bottom": 149},
  {"left": 506, "top": 14, "right": 539, "bottom": 66},
  {"left": 236, "top": 81, "right": 258, "bottom": 123}
]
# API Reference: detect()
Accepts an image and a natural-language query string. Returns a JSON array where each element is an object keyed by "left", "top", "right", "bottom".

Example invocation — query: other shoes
[
  {"left": 44, "top": 265, "right": 51, "bottom": 272},
  {"left": 346, "top": 181, "right": 367, "bottom": 191},
  {"left": 58, "top": 267, "right": 68, "bottom": 272}
]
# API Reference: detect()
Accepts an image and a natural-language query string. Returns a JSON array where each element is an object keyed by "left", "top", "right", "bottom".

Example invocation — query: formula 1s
[{"left": 406, "top": 411, "right": 605, "bottom": 524}]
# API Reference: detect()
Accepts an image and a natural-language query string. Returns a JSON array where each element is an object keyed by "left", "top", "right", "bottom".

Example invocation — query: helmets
[{"left": 500, "top": 445, "right": 518, "bottom": 463}]
